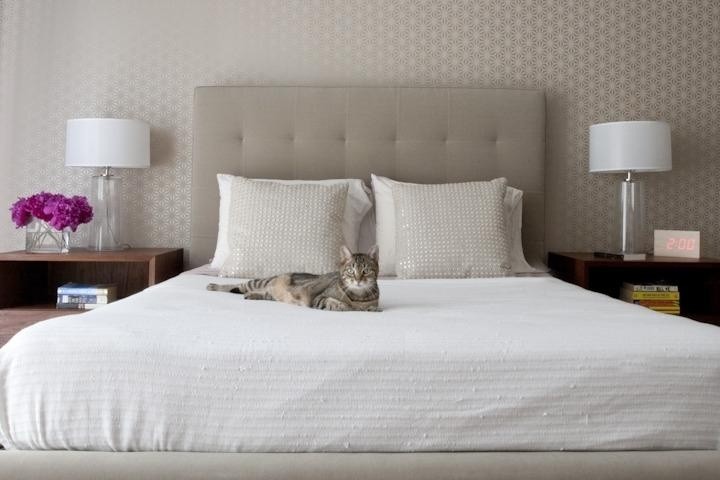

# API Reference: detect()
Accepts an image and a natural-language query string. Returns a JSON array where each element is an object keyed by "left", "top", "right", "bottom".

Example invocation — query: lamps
[
  {"left": 65, "top": 117, "right": 151, "bottom": 252},
  {"left": 589, "top": 120, "right": 672, "bottom": 255}
]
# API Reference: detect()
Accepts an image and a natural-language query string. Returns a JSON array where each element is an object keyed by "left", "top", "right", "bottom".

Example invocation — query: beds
[{"left": 0, "top": 85, "right": 720, "bottom": 480}]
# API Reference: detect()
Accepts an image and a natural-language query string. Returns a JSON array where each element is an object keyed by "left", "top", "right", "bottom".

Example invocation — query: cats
[{"left": 206, "top": 244, "right": 379, "bottom": 312}]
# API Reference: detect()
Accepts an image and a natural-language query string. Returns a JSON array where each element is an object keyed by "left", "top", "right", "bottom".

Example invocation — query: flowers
[{"left": 9, "top": 190, "right": 94, "bottom": 249}]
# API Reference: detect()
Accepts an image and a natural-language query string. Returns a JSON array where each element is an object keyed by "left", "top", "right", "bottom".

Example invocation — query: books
[
  {"left": 56, "top": 282, "right": 118, "bottom": 309},
  {"left": 621, "top": 283, "right": 680, "bottom": 315}
]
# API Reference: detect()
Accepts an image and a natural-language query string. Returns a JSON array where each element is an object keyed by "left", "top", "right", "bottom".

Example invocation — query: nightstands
[
  {"left": 547, "top": 251, "right": 720, "bottom": 327},
  {"left": 0, "top": 246, "right": 184, "bottom": 349}
]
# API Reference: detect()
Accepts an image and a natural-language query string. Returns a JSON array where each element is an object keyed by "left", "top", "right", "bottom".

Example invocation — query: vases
[{"left": 25, "top": 217, "right": 69, "bottom": 254}]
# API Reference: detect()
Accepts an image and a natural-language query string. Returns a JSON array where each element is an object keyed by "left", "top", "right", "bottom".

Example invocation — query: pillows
[
  {"left": 371, "top": 173, "right": 536, "bottom": 279},
  {"left": 208, "top": 173, "right": 373, "bottom": 279}
]
